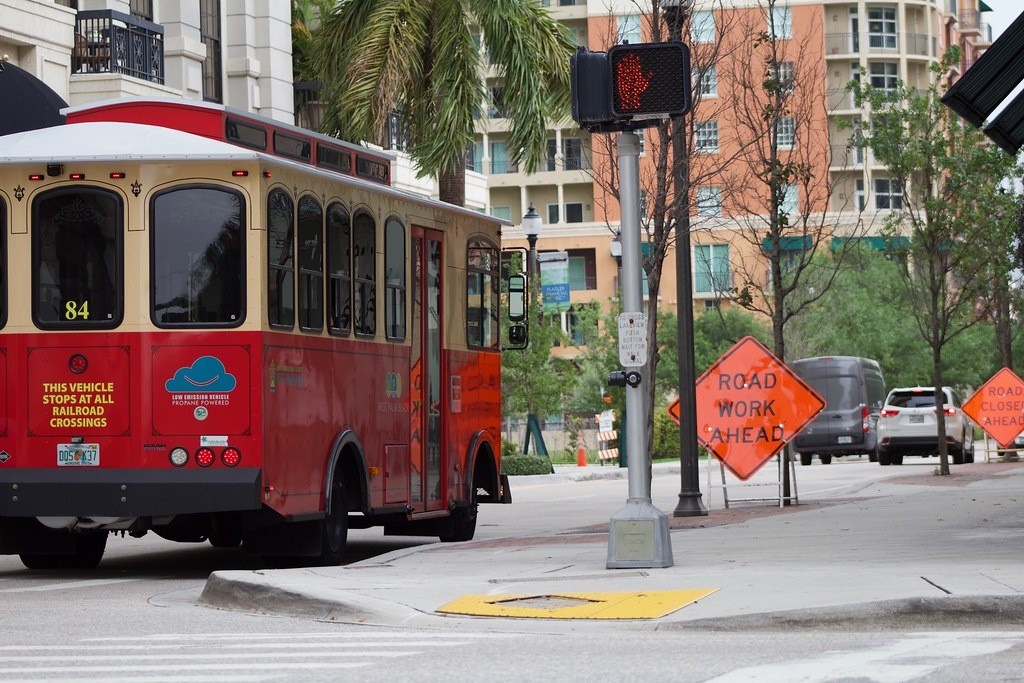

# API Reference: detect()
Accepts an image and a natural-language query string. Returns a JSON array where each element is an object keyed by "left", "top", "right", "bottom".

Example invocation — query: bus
[{"left": 0, "top": 95, "right": 530, "bottom": 575}]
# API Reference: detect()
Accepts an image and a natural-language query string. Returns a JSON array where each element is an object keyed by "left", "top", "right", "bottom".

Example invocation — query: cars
[{"left": 996, "top": 429, "right": 1024, "bottom": 456}]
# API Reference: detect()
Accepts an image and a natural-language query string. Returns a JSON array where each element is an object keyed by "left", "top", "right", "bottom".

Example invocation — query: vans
[{"left": 791, "top": 355, "right": 896, "bottom": 466}]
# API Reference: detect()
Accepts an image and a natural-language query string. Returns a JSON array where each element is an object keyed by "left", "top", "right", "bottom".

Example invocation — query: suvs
[{"left": 875, "top": 384, "right": 975, "bottom": 466}]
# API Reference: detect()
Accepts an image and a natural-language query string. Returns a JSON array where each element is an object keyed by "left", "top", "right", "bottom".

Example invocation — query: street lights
[
  {"left": 520, "top": 201, "right": 543, "bottom": 353},
  {"left": 655, "top": 1, "right": 709, "bottom": 518},
  {"left": 609, "top": 228, "right": 623, "bottom": 315}
]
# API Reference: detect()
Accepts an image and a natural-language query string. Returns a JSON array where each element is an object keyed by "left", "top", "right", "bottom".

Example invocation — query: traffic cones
[{"left": 575, "top": 433, "right": 588, "bottom": 467}]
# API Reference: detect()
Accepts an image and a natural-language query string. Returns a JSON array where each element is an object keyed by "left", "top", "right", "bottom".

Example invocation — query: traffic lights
[{"left": 607, "top": 39, "right": 691, "bottom": 119}]
